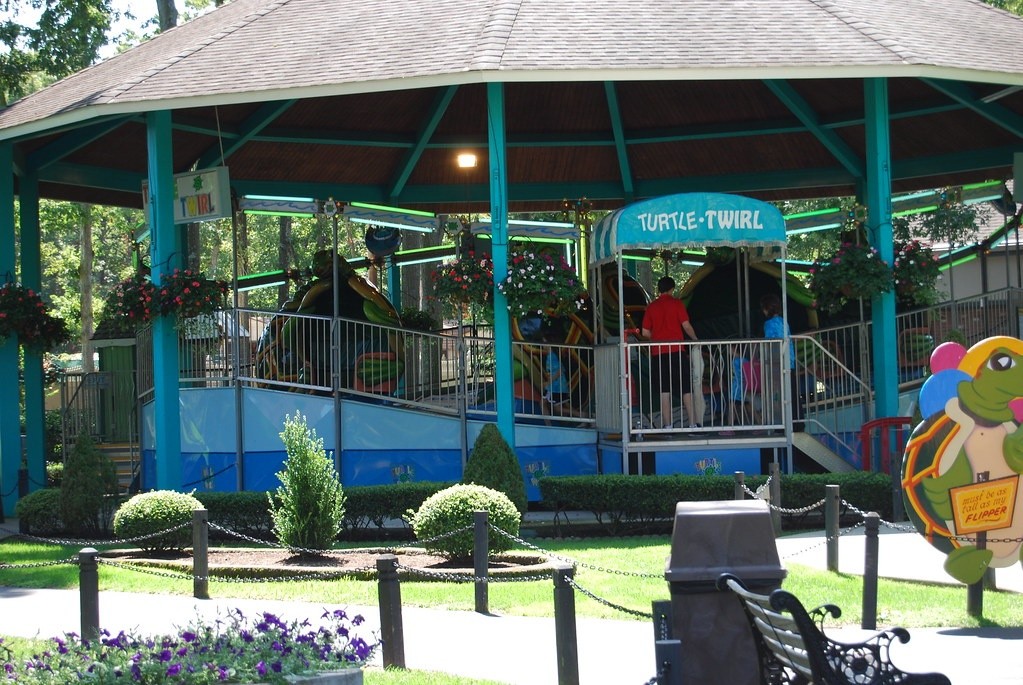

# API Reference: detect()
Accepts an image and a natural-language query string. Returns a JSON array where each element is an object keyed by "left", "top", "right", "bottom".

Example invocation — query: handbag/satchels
[{"left": 742, "top": 354, "right": 761, "bottom": 393}]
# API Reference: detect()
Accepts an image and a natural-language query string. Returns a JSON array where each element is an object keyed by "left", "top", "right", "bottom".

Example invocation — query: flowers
[
  {"left": 809, "top": 237, "right": 943, "bottom": 307},
  {"left": 100, "top": 267, "right": 229, "bottom": 329},
  {"left": 430, "top": 243, "right": 591, "bottom": 320},
  {"left": 0, "top": 281, "right": 72, "bottom": 353}
]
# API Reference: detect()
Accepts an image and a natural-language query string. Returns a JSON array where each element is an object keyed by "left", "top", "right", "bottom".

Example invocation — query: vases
[
  {"left": 896, "top": 281, "right": 913, "bottom": 295},
  {"left": 841, "top": 284, "right": 857, "bottom": 296}
]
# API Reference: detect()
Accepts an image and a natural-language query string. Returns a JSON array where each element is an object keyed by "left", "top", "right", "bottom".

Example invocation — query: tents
[{"left": 587, "top": 192, "right": 790, "bottom": 340}]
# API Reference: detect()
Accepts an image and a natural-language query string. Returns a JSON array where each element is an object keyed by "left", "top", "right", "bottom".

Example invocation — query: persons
[
  {"left": 760, "top": 296, "right": 806, "bottom": 435},
  {"left": 643, "top": 278, "right": 709, "bottom": 437},
  {"left": 541, "top": 332, "right": 588, "bottom": 428},
  {"left": 716, "top": 334, "right": 768, "bottom": 436}
]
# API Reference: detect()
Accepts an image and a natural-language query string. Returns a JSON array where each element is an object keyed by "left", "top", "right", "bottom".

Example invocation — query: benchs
[{"left": 714, "top": 572, "right": 954, "bottom": 684}]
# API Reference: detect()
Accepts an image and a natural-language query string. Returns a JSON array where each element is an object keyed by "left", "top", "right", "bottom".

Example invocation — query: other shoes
[
  {"left": 773, "top": 429, "right": 783, "bottom": 435},
  {"left": 689, "top": 423, "right": 709, "bottom": 437},
  {"left": 752, "top": 430, "right": 767, "bottom": 435},
  {"left": 718, "top": 431, "right": 735, "bottom": 435},
  {"left": 663, "top": 423, "right": 674, "bottom": 437}
]
